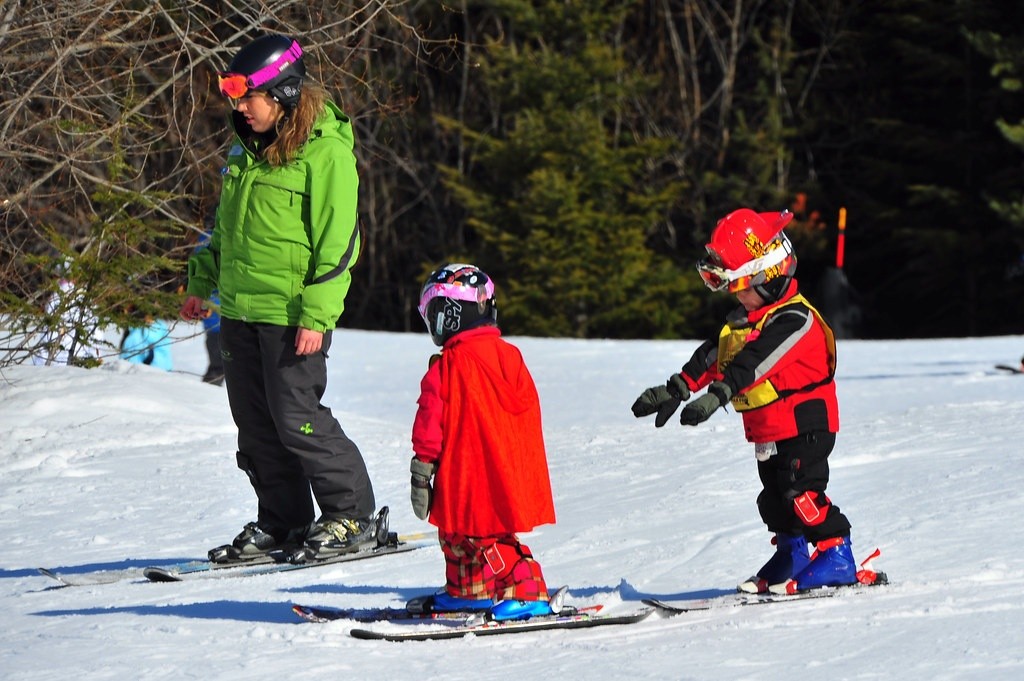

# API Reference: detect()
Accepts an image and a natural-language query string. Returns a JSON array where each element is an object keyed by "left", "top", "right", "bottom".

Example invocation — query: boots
[
  {"left": 756, "top": 528, "right": 810, "bottom": 585},
  {"left": 791, "top": 535, "right": 859, "bottom": 595}
]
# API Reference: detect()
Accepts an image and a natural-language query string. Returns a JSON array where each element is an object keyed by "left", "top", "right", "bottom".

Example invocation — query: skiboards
[
  {"left": 640, "top": 571, "right": 889, "bottom": 613},
  {"left": 37, "top": 531, "right": 440, "bottom": 586},
  {"left": 291, "top": 593, "right": 657, "bottom": 642}
]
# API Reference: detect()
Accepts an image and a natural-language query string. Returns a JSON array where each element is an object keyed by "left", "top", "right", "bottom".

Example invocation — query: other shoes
[
  {"left": 299, "top": 514, "right": 378, "bottom": 559},
  {"left": 486, "top": 600, "right": 557, "bottom": 622},
  {"left": 432, "top": 583, "right": 493, "bottom": 612},
  {"left": 232, "top": 520, "right": 316, "bottom": 559}
]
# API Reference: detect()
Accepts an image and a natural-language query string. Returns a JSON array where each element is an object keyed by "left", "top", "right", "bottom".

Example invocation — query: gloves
[
  {"left": 680, "top": 380, "right": 733, "bottom": 426},
  {"left": 631, "top": 373, "right": 690, "bottom": 428},
  {"left": 410, "top": 456, "right": 438, "bottom": 520}
]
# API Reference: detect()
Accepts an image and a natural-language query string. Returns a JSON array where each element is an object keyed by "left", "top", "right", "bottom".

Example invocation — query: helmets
[
  {"left": 418, "top": 262, "right": 498, "bottom": 346},
  {"left": 227, "top": 32, "right": 306, "bottom": 122},
  {"left": 705, "top": 208, "right": 798, "bottom": 304}
]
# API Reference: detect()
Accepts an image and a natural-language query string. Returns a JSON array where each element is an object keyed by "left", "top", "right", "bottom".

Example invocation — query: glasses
[
  {"left": 695, "top": 253, "right": 729, "bottom": 294},
  {"left": 216, "top": 71, "right": 249, "bottom": 100}
]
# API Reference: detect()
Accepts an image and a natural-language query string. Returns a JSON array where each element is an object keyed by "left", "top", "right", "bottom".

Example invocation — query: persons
[
  {"left": 190, "top": 226, "right": 230, "bottom": 386},
  {"left": 631, "top": 207, "right": 857, "bottom": 592},
  {"left": 110, "top": 271, "right": 173, "bottom": 371},
  {"left": 407, "top": 265, "right": 560, "bottom": 611},
  {"left": 43, "top": 254, "right": 101, "bottom": 343},
  {"left": 177, "top": 35, "right": 386, "bottom": 563}
]
